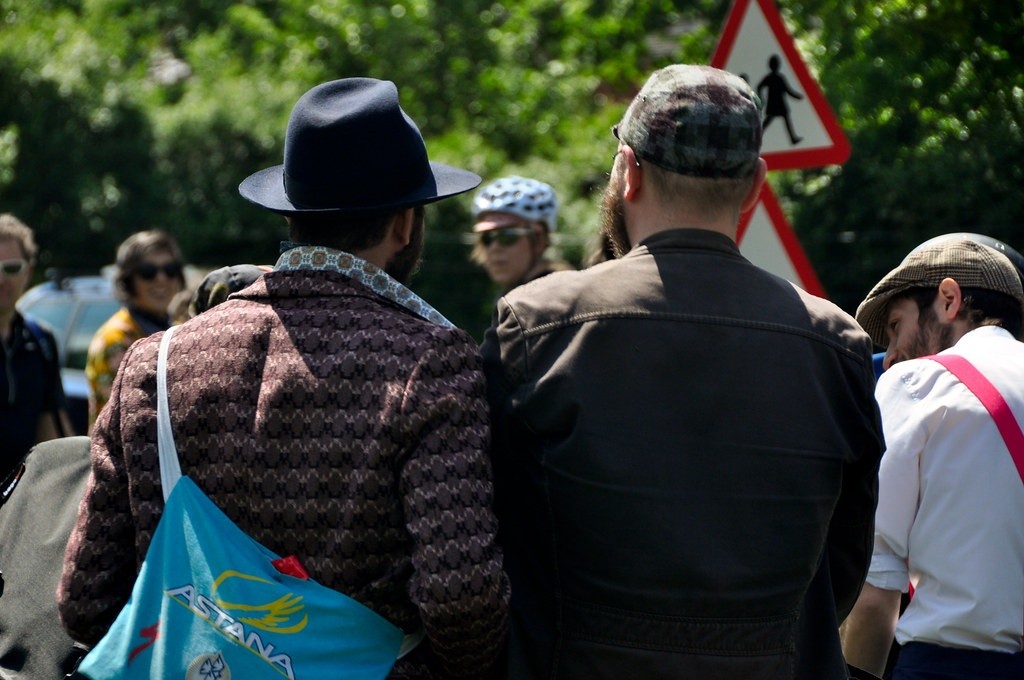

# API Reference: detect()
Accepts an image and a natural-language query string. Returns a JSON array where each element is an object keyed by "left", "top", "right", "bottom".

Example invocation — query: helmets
[{"left": 471, "top": 176, "right": 559, "bottom": 233}]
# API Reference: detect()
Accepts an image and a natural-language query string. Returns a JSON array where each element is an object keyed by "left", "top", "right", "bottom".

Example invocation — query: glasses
[
  {"left": 0, "top": 258, "right": 29, "bottom": 277},
  {"left": 481, "top": 228, "right": 530, "bottom": 247},
  {"left": 134, "top": 262, "right": 179, "bottom": 280}
]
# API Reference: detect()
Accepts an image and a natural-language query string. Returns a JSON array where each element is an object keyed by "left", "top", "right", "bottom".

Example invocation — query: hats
[
  {"left": 238, "top": 77, "right": 482, "bottom": 220},
  {"left": 855, "top": 240, "right": 1024, "bottom": 349},
  {"left": 472, "top": 213, "right": 520, "bottom": 233},
  {"left": 187, "top": 263, "right": 276, "bottom": 318},
  {"left": 612, "top": 64, "right": 764, "bottom": 178}
]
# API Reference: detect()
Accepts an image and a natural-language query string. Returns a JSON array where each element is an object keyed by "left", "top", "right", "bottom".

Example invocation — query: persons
[
  {"left": 0, "top": 264, "right": 275, "bottom": 680},
  {"left": 836, "top": 231, "right": 1024, "bottom": 680},
  {"left": 0, "top": 214, "right": 67, "bottom": 506},
  {"left": 60, "top": 77, "right": 512, "bottom": 680},
  {"left": 87, "top": 229, "right": 194, "bottom": 434},
  {"left": 471, "top": 176, "right": 575, "bottom": 296},
  {"left": 478, "top": 63, "right": 887, "bottom": 680}
]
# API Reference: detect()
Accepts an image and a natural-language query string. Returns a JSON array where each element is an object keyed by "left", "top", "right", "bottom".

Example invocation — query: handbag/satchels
[{"left": 77, "top": 326, "right": 426, "bottom": 680}]
